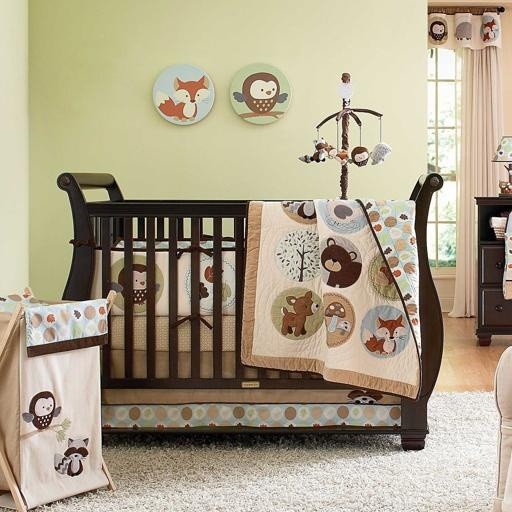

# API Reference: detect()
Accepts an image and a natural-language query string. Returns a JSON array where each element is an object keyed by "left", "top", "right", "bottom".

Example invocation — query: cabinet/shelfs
[{"left": 474, "top": 196, "right": 512, "bottom": 346}]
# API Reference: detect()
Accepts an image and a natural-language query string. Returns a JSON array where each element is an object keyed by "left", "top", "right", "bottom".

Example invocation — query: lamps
[{"left": 490, "top": 136, "right": 512, "bottom": 197}]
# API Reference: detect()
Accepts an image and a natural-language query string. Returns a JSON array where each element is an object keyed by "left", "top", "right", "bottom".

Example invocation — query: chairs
[{"left": 493, "top": 344, "right": 512, "bottom": 512}]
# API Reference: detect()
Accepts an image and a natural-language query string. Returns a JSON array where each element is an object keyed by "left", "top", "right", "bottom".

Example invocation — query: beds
[{"left": 56, "top": 171, "right": 443, "bottom": 451}]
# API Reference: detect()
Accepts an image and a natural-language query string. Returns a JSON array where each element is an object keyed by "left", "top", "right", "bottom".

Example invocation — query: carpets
[{"left": 0, "top": 388, "right": 501, "bottom": 512}]
